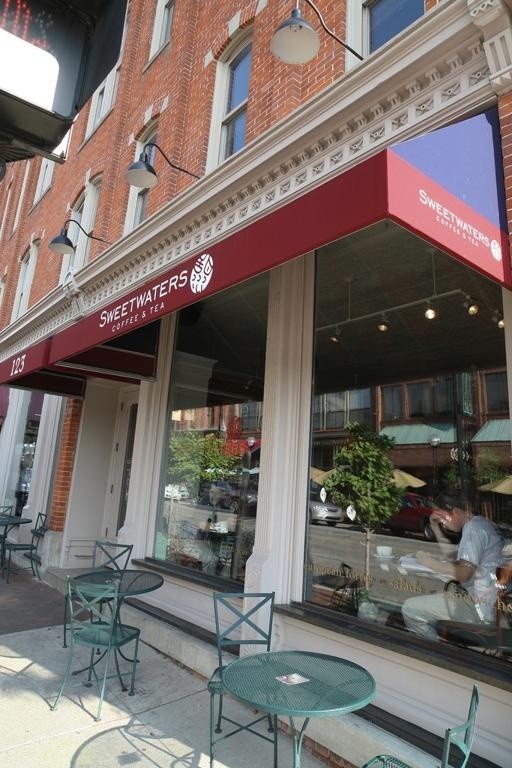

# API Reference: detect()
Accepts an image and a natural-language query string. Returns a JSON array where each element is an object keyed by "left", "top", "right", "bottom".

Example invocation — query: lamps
[
  {"left": 328, "top": 287, "right": 504, "bottom": 344},
  {"left": 45, "top": 218, "right": 112, "bottom": 260},
  {"left": 265, "top": 1, "right": 366, "bottom": 78},
  {"left": 125, "top": 140, "right": 199, "bottom": 195}
]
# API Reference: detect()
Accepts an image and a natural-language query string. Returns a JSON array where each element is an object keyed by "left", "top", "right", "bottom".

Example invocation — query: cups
[
  {"left": 216, "top": 520, "right": 228, "bottom": 534},
  {"left": 376, "top": 546, "right": 392, "bottom": 556}
]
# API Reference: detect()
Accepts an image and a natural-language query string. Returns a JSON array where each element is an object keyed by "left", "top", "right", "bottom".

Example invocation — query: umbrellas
[
  {"left": 311, "top": 466, "right": 426, "bottom": 494},
  {"left": 310, "top": 466, "right": 325, "bottom": 479},
  {"left": 477, "top": 474, "right": 511, "bottom": 494}
]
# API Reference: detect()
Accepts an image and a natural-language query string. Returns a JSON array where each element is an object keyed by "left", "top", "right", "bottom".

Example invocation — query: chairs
[
  {"left": 360, "top": 680, "right": 481, "bottom": 768},
  {"left": 206, "top": 591, "right": 279, "bottom": 768},
  {"left": 434, "top": 560, "right": 512, "bottom": 658},
  {"left": 50, "top": 539, "right": 165, "bottom": 722},
  {"left": 0, "top": 504, "right": 48, "bottom": 584}
]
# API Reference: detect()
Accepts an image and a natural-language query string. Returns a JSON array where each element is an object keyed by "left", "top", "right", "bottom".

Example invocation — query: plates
[{"left": 373, "top": 554, "right": 396, "bottom": 561}]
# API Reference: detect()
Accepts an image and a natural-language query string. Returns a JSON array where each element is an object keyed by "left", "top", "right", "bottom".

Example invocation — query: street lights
[
  {"left": 244, "top": 436, "right": 256, "bottom": 476},
  {"left": 427, "top": 435, "right": 443, "bottom": 488}
]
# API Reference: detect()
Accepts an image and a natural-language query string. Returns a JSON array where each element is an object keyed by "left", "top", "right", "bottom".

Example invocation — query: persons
[
  {"left": 174, "top": 480, "right": 230, "bottom": 575},
  {"left": 401, "top": 487, "right": 511, "bottom": 643}
]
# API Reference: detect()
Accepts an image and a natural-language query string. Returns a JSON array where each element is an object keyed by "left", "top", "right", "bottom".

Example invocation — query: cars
[{"left": 163, "top": 467, "right": 458, "bottom": 545}]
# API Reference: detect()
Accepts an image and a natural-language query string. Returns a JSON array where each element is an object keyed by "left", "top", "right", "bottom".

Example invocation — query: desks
[{"left": 222, "top": 650, "right": 376, "bottom": 767}]
